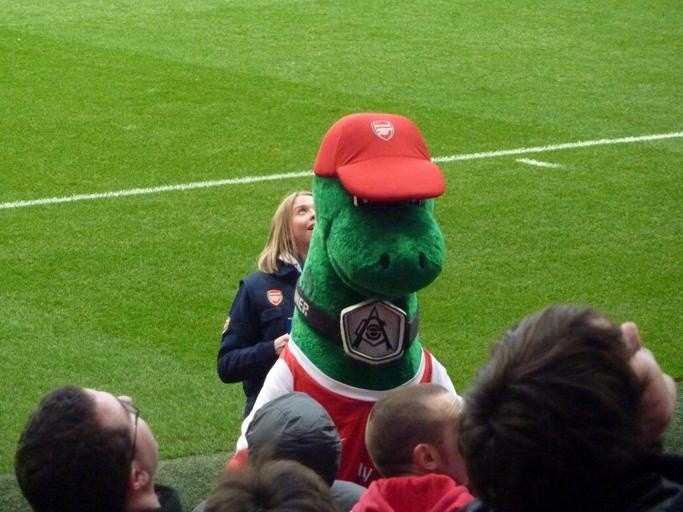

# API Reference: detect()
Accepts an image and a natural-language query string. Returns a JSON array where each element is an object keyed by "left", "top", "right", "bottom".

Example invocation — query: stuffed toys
[{"left": 234, "top": 114, "right": 467, "bottom": 484}]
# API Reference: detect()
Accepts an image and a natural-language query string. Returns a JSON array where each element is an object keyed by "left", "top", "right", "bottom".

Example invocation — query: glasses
[{"left": 113, "top": 392, "right": 139, "bottom": 458}]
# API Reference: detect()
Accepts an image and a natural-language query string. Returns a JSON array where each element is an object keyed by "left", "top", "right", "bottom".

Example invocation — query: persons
[
  {"left": 194, "top": 307, "right": 683, "bottom": 512},
  {"left": 15, "top": 385, "right": 185, "bottom": 512},
  {"left": 216, "top": 191, "right": 316, "bottom": 420}
]
